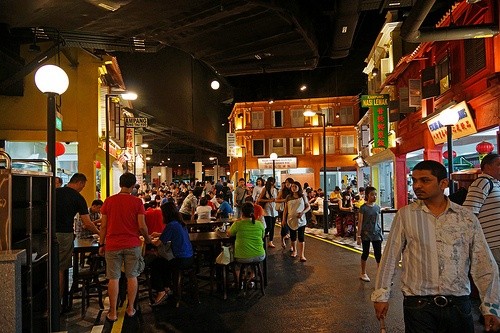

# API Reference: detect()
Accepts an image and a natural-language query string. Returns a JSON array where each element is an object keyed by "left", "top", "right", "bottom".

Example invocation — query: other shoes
[
  {"left": 290, "top": 252, "right": 297, "bottom": 257},
  {"left": 479, "top": 315, "right": 484, "bottom": 321},
  {"left": 165, "top": 288, "right": 171, "bottom": 294},
  {"left": 42, "top": 311, "right": 48, "bottom": 317},
  {"left": 150, "top": 293, "right": 168, "bottom": 307},
  {"left": 391, "top": 282, "right": 393, "bottom": 284},
  {"left": 248, "top": 281, "right": 255, "bottom": 288},
  {"left": 290, "top": 248, "right": 293, "bottom": 251},
  {"left": 232, "top": 282, "right": 244, "bottom": 289},
  {"left": 360, "top": 273, "right": 370, "bottom": 281},
  {"left": 300, "top": 257, "right": 306, "bottom": 261},
  {"left": 284, "top": 235, "right": 289, "bottom": 240},
  {"left": 282, "top": 243, "right": 286, "bottom": 248},
  {"left": 287, "top": 233, "right": 290, "bottom": 237},
  {"left": 268, "top": 244, "right": 275, "bottom": 248}
]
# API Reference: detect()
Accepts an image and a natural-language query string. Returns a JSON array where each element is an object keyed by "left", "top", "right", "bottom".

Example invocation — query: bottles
[{"left": 222, "top": 222, "right": 230, "bottom": 231}]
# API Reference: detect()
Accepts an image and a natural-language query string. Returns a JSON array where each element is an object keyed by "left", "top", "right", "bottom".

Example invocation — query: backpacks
[{"left": 448, "top": 176, "right": 493, "bottom": 206}]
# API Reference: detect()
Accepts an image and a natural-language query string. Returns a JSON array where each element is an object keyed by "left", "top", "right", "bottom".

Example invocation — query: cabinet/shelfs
[{"left": 0, "top": 151, "right": 52, "bottom": 333}]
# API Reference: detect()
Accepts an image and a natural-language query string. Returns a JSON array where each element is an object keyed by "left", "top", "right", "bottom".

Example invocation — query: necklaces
[{"left": 120, "top": 192, "right": 130, "bottom": 194}]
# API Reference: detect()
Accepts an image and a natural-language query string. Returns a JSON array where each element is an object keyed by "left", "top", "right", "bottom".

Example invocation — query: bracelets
[
  {"left": 357, "top": 236, "right": 361, "bottom": 238},
  {"left": 99, "top": 244, "right": 104, "bottom": 247}
]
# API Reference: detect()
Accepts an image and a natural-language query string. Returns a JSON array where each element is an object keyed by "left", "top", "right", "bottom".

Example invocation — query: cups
[{"left": 228, "top": 213, "right": 232, "bottom": 220}]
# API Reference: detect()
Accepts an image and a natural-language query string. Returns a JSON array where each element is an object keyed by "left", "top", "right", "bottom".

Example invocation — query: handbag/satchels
[
  {"left": 303, "top": 196, "right": 312, "bottom": 223},
  {"left": 157, "top": 243, "right": 174, "bottom": 261},
  {"left": 256, "top": 188, "right": 268, "bottom": 209}
]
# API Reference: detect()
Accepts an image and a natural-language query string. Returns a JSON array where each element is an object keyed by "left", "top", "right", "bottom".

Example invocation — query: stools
[{"left": 68, "top": 262, "right": 265, "bottom": 313}]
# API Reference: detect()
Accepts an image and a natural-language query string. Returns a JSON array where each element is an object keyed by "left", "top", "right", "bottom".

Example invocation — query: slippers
[
  {"left": 107, "top": 311, "right": 117, "bottom": 321},
  {"left": 127, "top": 308, "right": 136, "bottom": 320}
]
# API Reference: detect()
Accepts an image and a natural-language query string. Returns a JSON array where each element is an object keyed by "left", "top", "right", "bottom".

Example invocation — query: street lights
[
  {"left": 34, "top": 65, "right": 69, "bottom": 332},
  {"left": 233, "top": 145, "right": 246, "bottom": 186},
  {"left": 104, "top": 92, "right": 139, "bottom": 198},
  {"left": 134, "top": 143, "right": 149, "bottom": 175},
  {"left": 438, "top": 108, "right": 460, "bottom": 196},
  {"left": 208, "top": 157, "right": 219, "bottom": 184},
  {"left": 158, "top": 172, "right": 161, "bottom": 186},
  {"left": 303, "top": 111, "right": 328, "bottom": 234},
  {"left": 269, "top": 153, "right": 278, "bottom": 180},
  {"left": 226, "top": 172, "right": 230, "bottom": 181}
]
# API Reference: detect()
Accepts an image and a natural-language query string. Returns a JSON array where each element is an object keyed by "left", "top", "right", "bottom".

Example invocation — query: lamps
[{"left": 29, "top": 30, "right": 42, "bottom": 53}]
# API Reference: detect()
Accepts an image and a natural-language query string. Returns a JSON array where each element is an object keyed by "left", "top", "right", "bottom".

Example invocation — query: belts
[{"left": 406, "top": 295, "right": 469, "bottom": 307}]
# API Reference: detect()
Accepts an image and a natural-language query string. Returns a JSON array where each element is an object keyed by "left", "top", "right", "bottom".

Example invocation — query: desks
[
  {"left": 185, "top": 216, "right": 237, "bottom": 233},
  {"left": 73, "top": 230, "right": 232, "bottom": 298}
]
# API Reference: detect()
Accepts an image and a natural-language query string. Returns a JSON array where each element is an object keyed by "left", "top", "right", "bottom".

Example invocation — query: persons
[
  {"left": 53, "top": 173, "right": 101, "bottom": 317},
  {"left": 150, "top": 201, "right": 195, "bottom": 306},
  {"left": 462, "top": 153, "right": 500, "bottom": 262},
  {"left": 54, "top": 172, "right": 364, "bottom": 333},
  {"left": 356, "top": 186, "right": 384, "bottom": 281},
  {"left": 98, "top": 173, "right": 153, "bottom": 321},
  {"left": 371, "top": 159, "right": 500, "bottom": 333}
]
